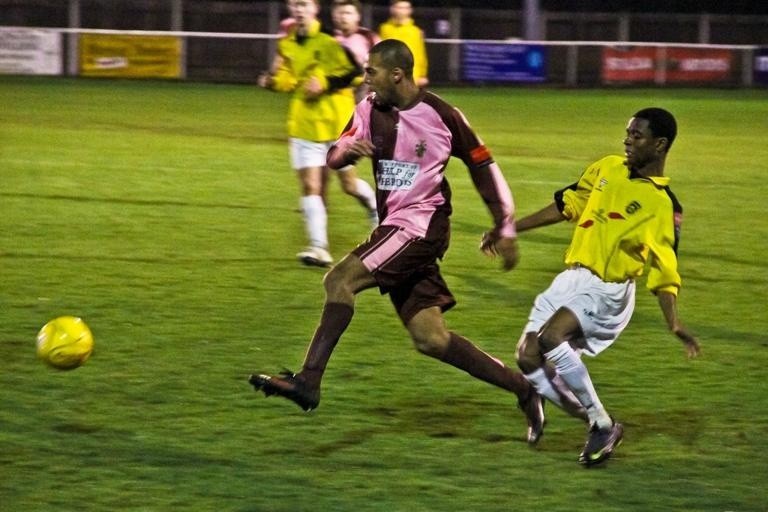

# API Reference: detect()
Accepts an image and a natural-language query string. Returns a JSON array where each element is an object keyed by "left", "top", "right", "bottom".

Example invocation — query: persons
[
  {"left": 257, "top": 0, "right": 376, "bottom": 268},
  {"left": 380, "top": 1, "right": 428, "bottom": 88},
  {"left": 321, "top": 3, "right": 376, "bottom": 218},
  {"left": 248, "top": 38, "right": 547, "bottom": 446},
  {"left": 479, "top": 108, "right": 700, "bottom": 468}
]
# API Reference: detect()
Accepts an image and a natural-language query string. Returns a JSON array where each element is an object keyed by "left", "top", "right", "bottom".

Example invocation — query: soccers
[{"left": 35, "top": 316, "right": 94, "bottom": 369}]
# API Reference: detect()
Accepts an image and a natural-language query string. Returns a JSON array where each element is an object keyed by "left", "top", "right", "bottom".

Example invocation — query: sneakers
[
  {"left": 249, "top": 370, "right": 320, "bottom": 412},
  {"left": 579, "top": 420, "right": 623, "bottom": 466},
  {"left": 296, "top": 247, "right": 332, "bottom": 267},
  {"left": 519, "top": 388, "right": 547, "bottom": 446},
  {"left": 370, "top": 203, "right": 382, "bottom": 229}
]
